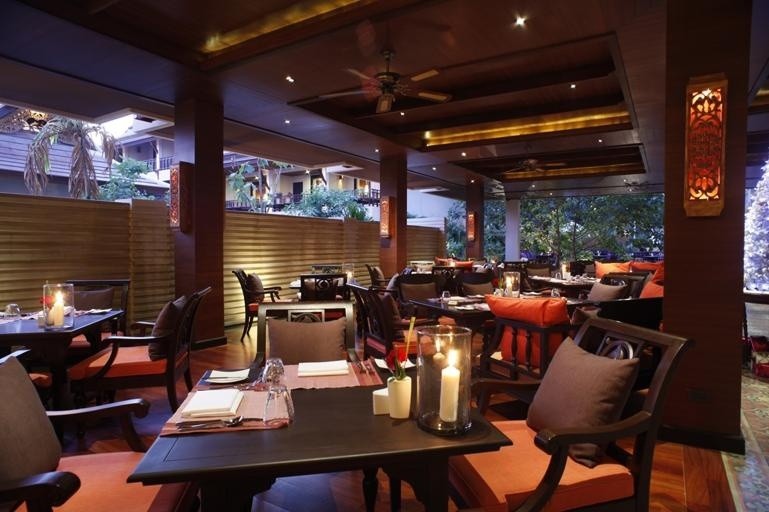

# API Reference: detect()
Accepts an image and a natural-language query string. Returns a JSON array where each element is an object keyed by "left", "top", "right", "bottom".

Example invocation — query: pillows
[
  {"left": 399, "top": 282, "right": 437, "bottom": 302},
  {"left": 653, "top": 262, "right": 664, "bottom": 280},
  {"left": 641, "top": 281, "right": 664, "bottom": 298},
  {"left": 455, "top": 261, "right": 472, "bottom": 268},
  {"left": 588, "top": 282, "right": 628, "bottom": 303},
  {"left": 372, "top": 266, "right": 385, "bottom": 285},
  {"left": 267, "top": 318, "right": 348, "bottom": 364},
  {"left": 463, "top": 281, "right": 494, "bottom": 296},
  {"left": 248, "top": 273, "right": 265, "bottom": 304},
  {"left": 73, "top": 288, "right": 115, "bottom": 310},
  {"left": 595, "top": 261, "right": 631, "bottom": 278},
  {"left": 434, "top": 257, "right": 451, "bottom": 265},
  {"left": 414, "top": 264, "right": 433, "bottom": 271},
  {"left": 527, "top": 266, "right": 550, "bottom": 277},
  {"left": 485, "top": 293, "right": 569, "bottom": 367},
  {"left": 633, "top": 262, "right": 658, "bottom": 272},
  {"left": 149, "top": 295, "right": 187, "bottom": 360},
  {"left": 526, "top": 338, "right": 639, "bottom": 468}
]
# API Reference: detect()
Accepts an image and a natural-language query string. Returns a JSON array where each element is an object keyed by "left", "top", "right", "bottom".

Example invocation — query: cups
[
  {"left": 263, "top": 384, "right": 296, "bottom": 428},
  {"left": 4, "top": 302, "right": 22, "bottom": 322},
  {"left": 415, "top": 323, "right": 474, "bottom": 436},
  {"left": 341, "top": 262, "right": 355, "bottom": 285},
  {"left": 441, "top": 291, "right": 450, "bottom": 303},
  {"left": 502, "top": 271, "right": 520, "bottom": 298},
  {"left": 555, "top": 271, "right": 561, "bottom": 279},
  {"left": 261, "top": 356, "right": 288, "bottom": 392},
  {"left": 43, "top": 283, "right": 75, "bottom": 331},
  {"left": 551, "top": 289, "right": 561, "bottom": 298}
]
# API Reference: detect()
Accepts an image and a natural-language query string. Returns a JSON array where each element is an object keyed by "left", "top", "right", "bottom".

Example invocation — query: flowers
[{"left": 384, "top": 344, "right": 409, "bottom": 379}]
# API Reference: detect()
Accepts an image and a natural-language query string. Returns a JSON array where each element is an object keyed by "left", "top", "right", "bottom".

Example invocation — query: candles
[
  {"left": 441, "top": 349, "right": 461, "bottom": 423},
  {"left": 52, "top": 292, "right": 64, "bottom": 324},
  {"left": 506, "top": 278, "right": 512, "bottom": 296}
]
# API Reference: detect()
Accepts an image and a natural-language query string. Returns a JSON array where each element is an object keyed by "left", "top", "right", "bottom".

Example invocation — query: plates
[{"left": 374, "top": 357, "right": 416, "bottom": 370}]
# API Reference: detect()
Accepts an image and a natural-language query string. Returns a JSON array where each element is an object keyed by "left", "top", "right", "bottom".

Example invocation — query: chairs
[
  {"left": 67, "top": 287, "right": 212, "bottom": 450},
  {"left": 407, "top": 260, "right": 436, "bottom": 272},
  {"left": 599, "top": 274, "right": 644, "bottom": 299},
  {"left": 249, "top": 301, "right": 358, "bottom": 371},
  {"left": 526, "top": 264, "right": 551, "bottom": 285},
  {"left": 232, "top": 268, "right": 292, "bottom": 341},
  {"left": 468, "top": 258, "right": 486, "bottom": 263},
  {"left": 345, "top": 282, "right": 436, "bottom": 364},
  {"left": 433, "top": 266, "right": 464, "bottom": 296},
  {"left": 365, "top": 263, "right": 390, "bottom": 291},
  {"left": 483, "top": 319, "right": 569, "bottom": 380},
  {"left": 570, "top": 260, "right": 595, "bottom": 278},
  {"left": 66, "top": 280, "right": 131, "bottom": 347},
  {"left": 1, "top": 357, "right": 202, "bottom": 512},
  {"left": 312, "top": 264, "right": 343, "bottom": 280},
  {"left": 1, "top": 346, "right": 52, "bottom": 394},
  {"left": 643, "top": 256, "right": 658, "bottom": 262},
  {"left": 396, "top": 274, "right": 441, "bottom": 320},
  {"left": 450, "top": 309, "right": 695, "bottom": 512},
  {"left": 456, "top": 273, "right": 494, "bottom": 298},
  {"left": 498, "top": 260, "right": 528, "bottom": 276},
  {"left": 299, "top": 273, "right": 347, "bottom": 317},
  {"left": 599, "top": 297, "right": 663, "bottom": 330}
]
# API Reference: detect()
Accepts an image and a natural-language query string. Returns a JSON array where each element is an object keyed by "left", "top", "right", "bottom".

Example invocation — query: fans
[{"left": 316, "top": 50, "right": 452, "bottom": 113}]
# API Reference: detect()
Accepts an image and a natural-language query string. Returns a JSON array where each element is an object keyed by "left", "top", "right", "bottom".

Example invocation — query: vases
[{"left": 387, "top": 374, "right": 407, "bottom": 419}]
